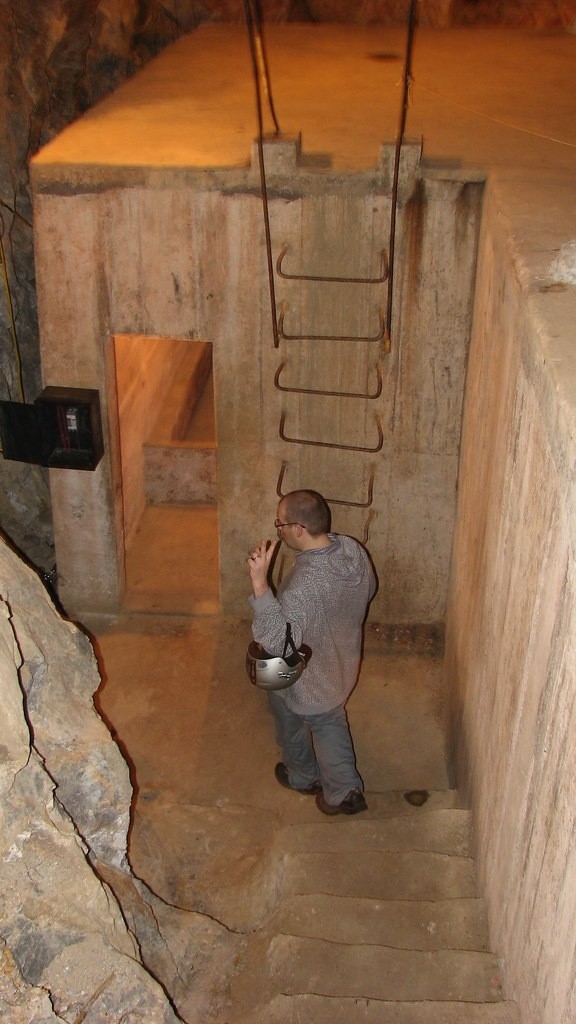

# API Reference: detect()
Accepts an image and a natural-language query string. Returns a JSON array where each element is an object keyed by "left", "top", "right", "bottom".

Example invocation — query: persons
[{"left": 245, "top": 490, "right": 377, "bottom": 816}]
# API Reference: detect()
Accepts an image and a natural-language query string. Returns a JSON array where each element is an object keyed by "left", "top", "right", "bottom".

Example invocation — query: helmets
[{"left": 246, "top": 639, "right": 312, "bottom": 690}]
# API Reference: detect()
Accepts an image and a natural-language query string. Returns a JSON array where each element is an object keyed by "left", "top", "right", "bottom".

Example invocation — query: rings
[{"left": 254, "top": 556, "right": 259, "bottom": 560}]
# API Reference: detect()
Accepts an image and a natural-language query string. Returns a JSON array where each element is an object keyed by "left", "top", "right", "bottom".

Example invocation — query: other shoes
[
  {"left": 315, "top": 788, "right": 368, "bottom": 816},
  {"left": 274, "top": 761, "right": 323, "bottom": 796}
]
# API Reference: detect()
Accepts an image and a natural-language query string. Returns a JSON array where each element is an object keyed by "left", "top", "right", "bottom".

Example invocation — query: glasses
[{"left": 273, "top": 519, "right": 305, "bottom": 530}]
